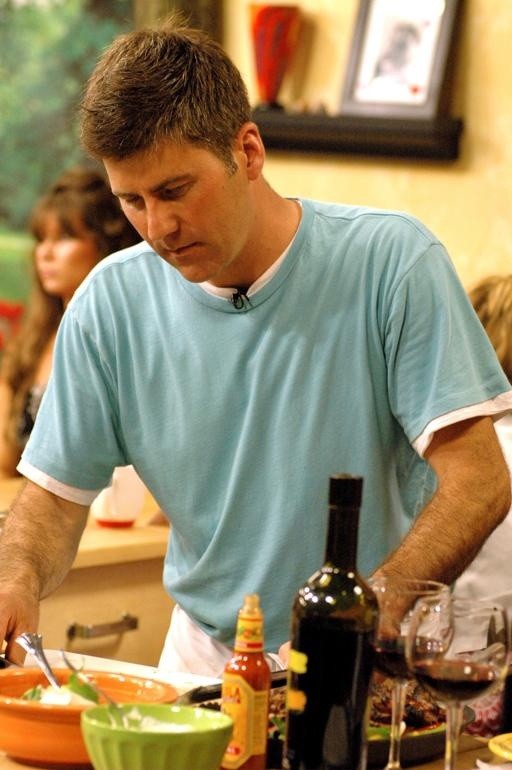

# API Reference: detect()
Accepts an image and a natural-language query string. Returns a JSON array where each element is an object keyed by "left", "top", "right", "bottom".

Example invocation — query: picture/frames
[{"left": 339, "top": 0, "right": 463, "bottom": 121}]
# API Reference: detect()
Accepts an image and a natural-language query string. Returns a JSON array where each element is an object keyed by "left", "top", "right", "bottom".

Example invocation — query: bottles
[
  {"left": 221, "top": 593, "right": 271, "bottom": 769},
  {"left": 277, "top": 475, "right": 379, "bottom": 769}
]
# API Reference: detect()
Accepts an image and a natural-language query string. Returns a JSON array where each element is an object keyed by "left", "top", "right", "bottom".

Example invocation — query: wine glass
[{"left": 367, "top": 574, "right": 506, "bottom": 769}]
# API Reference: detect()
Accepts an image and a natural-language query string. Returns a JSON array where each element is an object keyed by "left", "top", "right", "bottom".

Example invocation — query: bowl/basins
[
  {"left": 0, "top": 665, "right": 179, "bottom": 767},
  {"left": 80, "top": 702, "right": 238, "bottom": 770}
]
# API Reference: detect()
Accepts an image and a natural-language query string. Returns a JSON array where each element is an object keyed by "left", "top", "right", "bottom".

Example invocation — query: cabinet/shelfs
[{"left": 36, "top": 558, "right": 175, "bottom": 668}]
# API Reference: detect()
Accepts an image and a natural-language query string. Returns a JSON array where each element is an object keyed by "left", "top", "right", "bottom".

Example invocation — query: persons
[
  {"left": 1, "top": 26, "right": 511, "bottom": 693},
  {"left": 452, "top": 276, "right": 512, "bottom": 653},
  {"left": 1, "top": 169, "right": 143, "bottom": 476}
]
